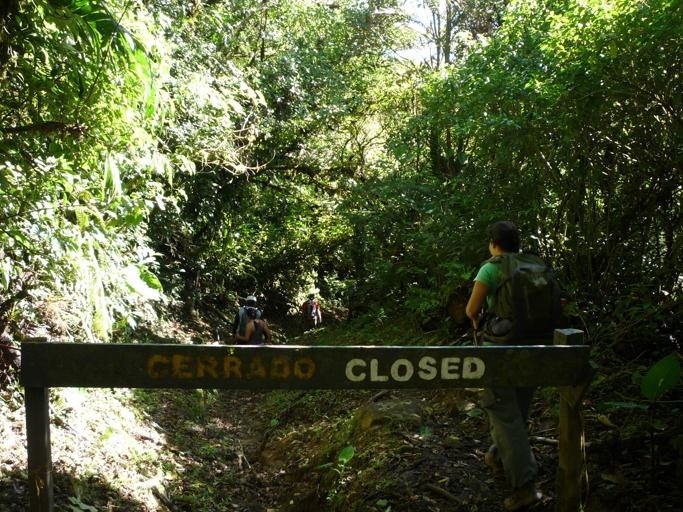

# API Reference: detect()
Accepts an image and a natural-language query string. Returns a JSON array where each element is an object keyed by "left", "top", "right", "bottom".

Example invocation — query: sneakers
[
  {"left": 484, "top": 452, "right": 501, "bottom": 475},
  {"left": 504, "top": 488, "right": 535, "bottom": 511}
]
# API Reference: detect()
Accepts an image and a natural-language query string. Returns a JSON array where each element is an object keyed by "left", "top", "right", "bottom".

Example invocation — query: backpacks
[{"left": 480, "top": 255, "right": 569, "bottom": 345}]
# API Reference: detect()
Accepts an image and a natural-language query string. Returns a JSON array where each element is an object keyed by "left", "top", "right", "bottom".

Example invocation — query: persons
[
  {"left": 299, "top": 293, "right": 323, "bottom": 329},
  {"left": 465, "top": 220, "right": 546, "bottom": 511},
  {"left": 235, "top": 307, "right": 272, "bottom": 345},
  {"left": 233, "top": 294, "right": 267, "bottom": 339}
]
